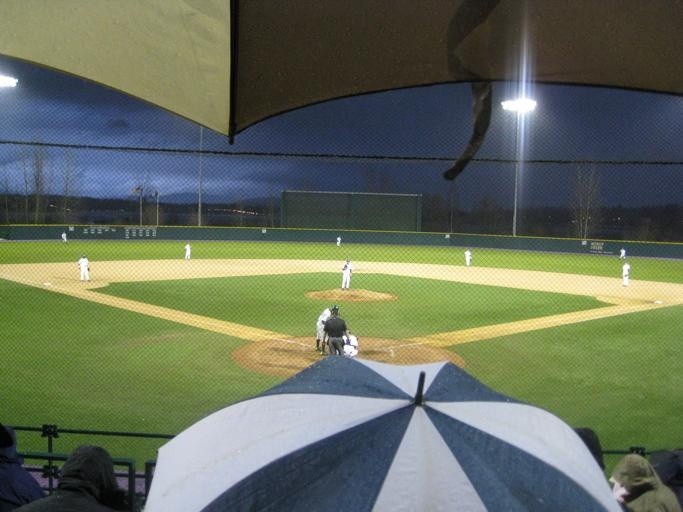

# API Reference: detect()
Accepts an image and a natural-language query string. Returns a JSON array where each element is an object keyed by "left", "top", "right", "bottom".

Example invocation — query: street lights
[{"left": 502, "top": 99, "right": 537, "bottom": 238}]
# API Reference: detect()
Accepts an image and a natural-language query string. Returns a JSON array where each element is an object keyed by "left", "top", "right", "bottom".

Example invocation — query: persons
[
  {"left": 575, "top": 428, "right": 606, "bottom": 471},
  {"left": 316, "top": 304, "right": 359, "bottom": 357},
  {"left": 78, "top": 255, "right": 90, "bottom": 281},
  {"left": 612, "top": 446, "right": 683, "bottom": 511},
  {"left": 342, "top": 259, "right": 352, "bottom": 290},
  {"left": 62, "top": 231, "right": 66, "bottom": 243},
  {"left": 619, "top": 247, "right": 626, "bottom": 260},
  {"left": 337, "top": 235, "right": 341, "bottom": 247},
  {"left": 1, "top": 424, "right": 143, "bottom": 511},
  {"left": 623, "top": 261, "right": 631, "bottom": 286},
  {"left": 185, "top": 242, "right": 192, "bottom": 260},
  {"left": 464, "top": 248, "right": 471, "bottom": 266}
]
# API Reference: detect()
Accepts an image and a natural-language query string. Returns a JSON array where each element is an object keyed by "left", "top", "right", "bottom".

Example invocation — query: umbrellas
[{"left": 145, "top": 356, "right": 624, "bottom": 512}]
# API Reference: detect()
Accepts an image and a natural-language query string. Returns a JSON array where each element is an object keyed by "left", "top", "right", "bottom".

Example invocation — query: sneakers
[{"left": 320, "top": 351, "right": 327, "bottom": 355}]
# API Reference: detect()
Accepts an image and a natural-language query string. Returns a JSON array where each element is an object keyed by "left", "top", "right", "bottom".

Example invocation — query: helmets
[{"left": 331, "top": 309, "right": 337, "bottom": 317}]
